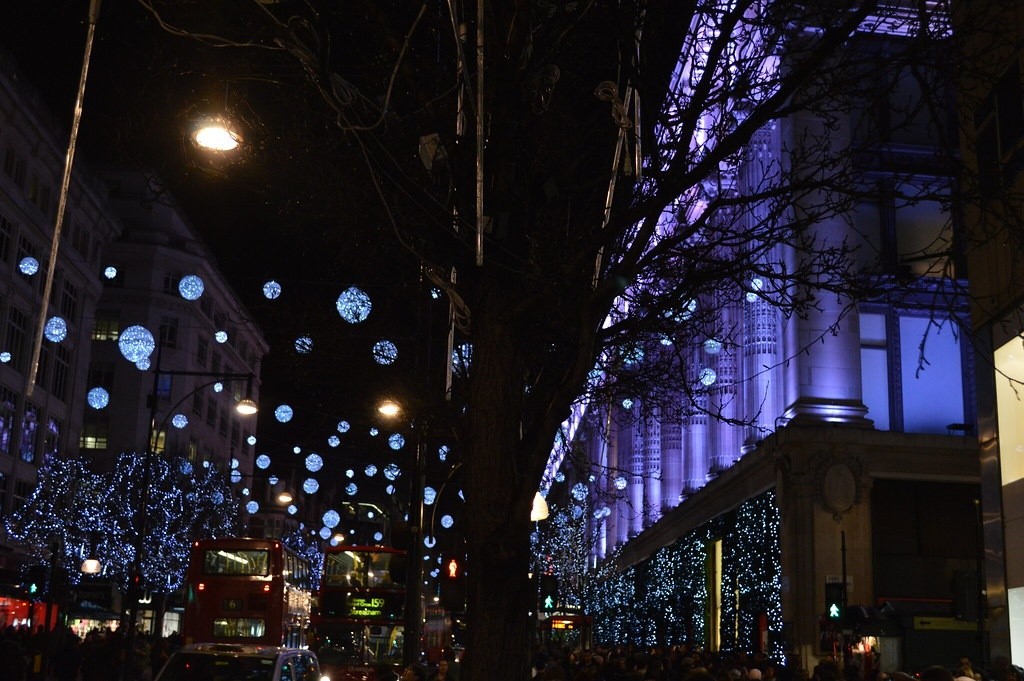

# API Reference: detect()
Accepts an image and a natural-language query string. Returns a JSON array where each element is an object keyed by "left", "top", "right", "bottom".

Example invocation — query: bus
[
  {"left": 182, "top": 537, "right": 318, "bottom": 651},
  {"left": 311, "top": 548, "right": 449, "bottom": 681}
]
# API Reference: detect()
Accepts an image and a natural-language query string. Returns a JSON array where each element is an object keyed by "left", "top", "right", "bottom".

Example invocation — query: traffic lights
[{"left": 825, "top": 582, "right": 842, "bottom": 621}]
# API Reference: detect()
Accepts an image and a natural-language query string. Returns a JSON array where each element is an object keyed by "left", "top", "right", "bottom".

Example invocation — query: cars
[{"left": 153, "top": 644, "right": 321, "bottom": 681}]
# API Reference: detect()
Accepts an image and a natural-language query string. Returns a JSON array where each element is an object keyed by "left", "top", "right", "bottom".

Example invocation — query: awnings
[{"left": 847, "top": 602, "right": 905, "bottom": 639}]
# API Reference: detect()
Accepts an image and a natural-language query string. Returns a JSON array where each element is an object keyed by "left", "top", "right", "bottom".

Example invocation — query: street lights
[{"left": 123, "top": 370, "right": 259, "bottom": 663}]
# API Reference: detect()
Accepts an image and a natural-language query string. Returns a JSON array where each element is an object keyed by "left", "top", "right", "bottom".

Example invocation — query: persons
[
  {"left": 531, "top": 641, "right": 1024, "bottom": 681},
  {"left": 375, "top": 657, "right": 456, "bottom": 681},
  {"left": 350, "top": 571, "right": 361, "bottom": 586},
  {"left": 0, "top": 624, "right": 184, "bottom": 681}
]
